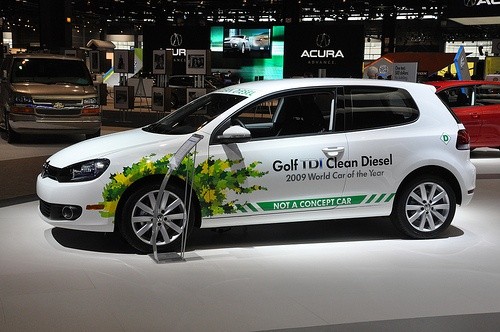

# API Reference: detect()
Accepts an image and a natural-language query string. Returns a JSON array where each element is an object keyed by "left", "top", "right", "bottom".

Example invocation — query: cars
[
  {"left": 423, "top": 80, "right": 500, "bottom": 151},
  {"left": 250, "top": 34, "right": 269, "bottom": 51},
  {"left": 164, "top": 74, "right": 226, "bottom": 110},
  {"left": 4, "top": 53, "right": 101, "bottom": 144},
  {"left": 224, "top": 35, "right": 251, "bottom": 54},
  {"left": 36, "top": 78, "right": 476, "bottom": 253}
]
